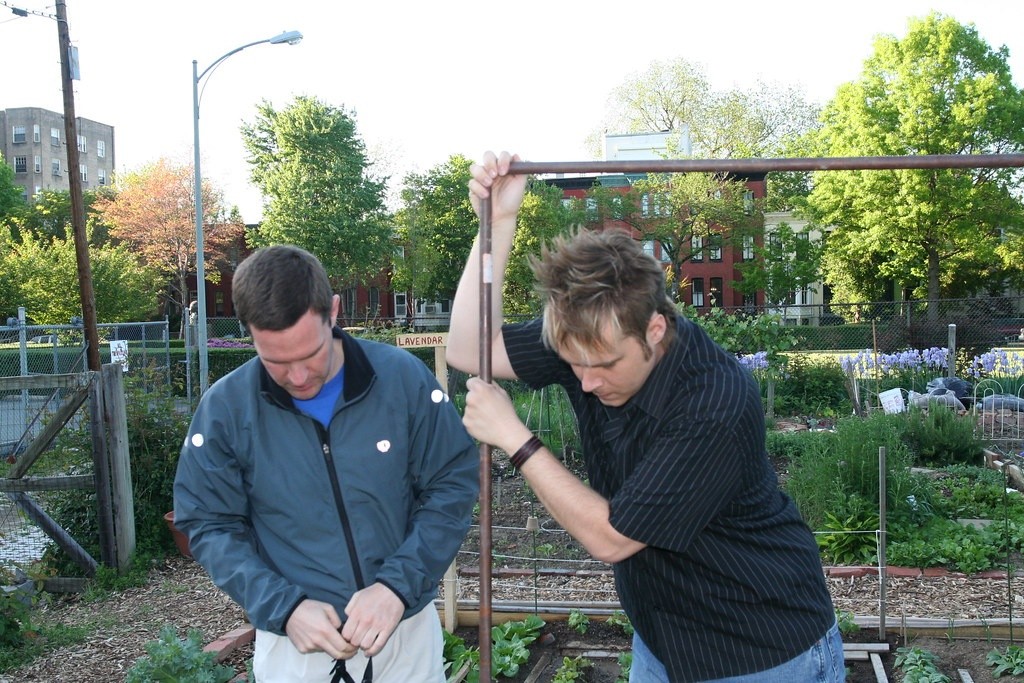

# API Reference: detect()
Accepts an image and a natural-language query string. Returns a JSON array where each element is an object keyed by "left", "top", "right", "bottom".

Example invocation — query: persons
[
  {"left": 446, "top": 151, "right": 847, "bottom": 683},
  {"left": 174, "top": 246, "right": 479, "bottom": 682}
]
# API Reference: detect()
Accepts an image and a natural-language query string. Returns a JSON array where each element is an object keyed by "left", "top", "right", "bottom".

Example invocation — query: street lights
[{"left": 194, "top": 30, "right": 304, "bottom": 397}]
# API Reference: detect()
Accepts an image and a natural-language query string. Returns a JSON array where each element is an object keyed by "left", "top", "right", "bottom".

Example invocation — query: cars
[{"left": 28, "top": 336, "right": 60, "bottom": 344}]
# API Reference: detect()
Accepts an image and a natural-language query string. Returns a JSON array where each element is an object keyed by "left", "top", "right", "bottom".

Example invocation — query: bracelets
[{"left": 509, "top": 435, "right": 544, "bottom": 469}]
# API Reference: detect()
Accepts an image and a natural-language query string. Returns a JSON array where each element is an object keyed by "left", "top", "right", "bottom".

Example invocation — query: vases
[{"left": 166, "top": 511, "right": 192, "bottom": 554}]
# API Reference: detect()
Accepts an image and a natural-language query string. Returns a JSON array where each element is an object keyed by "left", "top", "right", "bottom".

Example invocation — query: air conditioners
[{"left": 425, "top": 305, "right": 436, "bottom": 313}]
